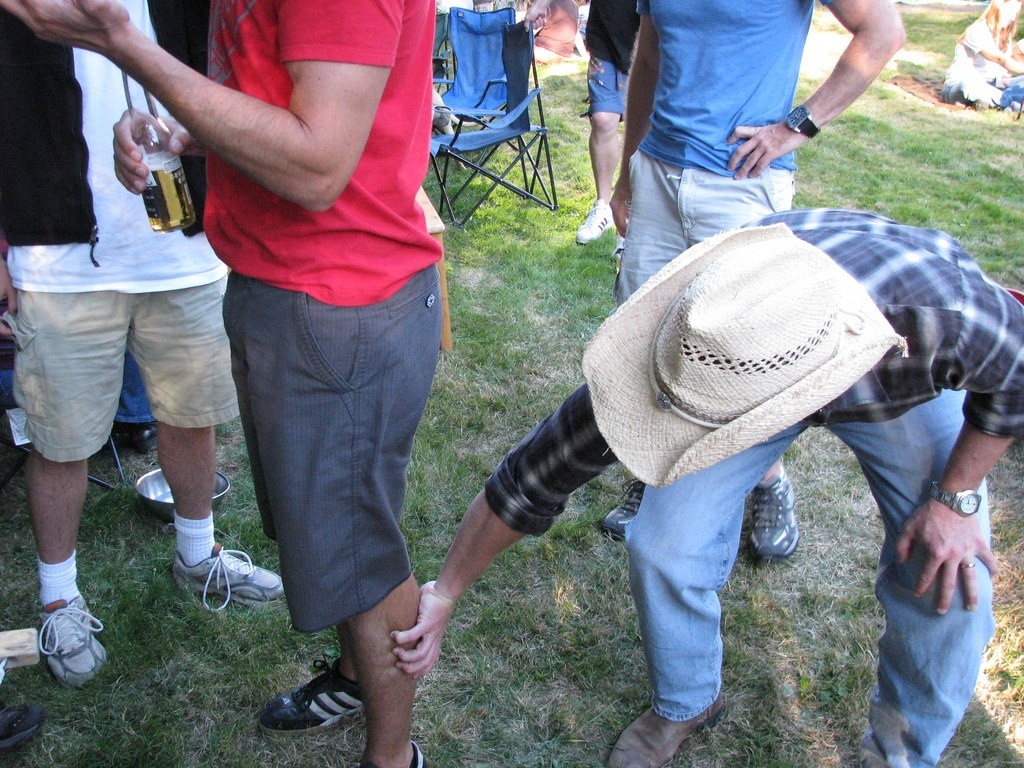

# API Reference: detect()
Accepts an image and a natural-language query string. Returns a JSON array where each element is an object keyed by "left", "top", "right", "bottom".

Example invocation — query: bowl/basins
[{"left": 135, "top": 467, "right": 231, "bottom": 523}]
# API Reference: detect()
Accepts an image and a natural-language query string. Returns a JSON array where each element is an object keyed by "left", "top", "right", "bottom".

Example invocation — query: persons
[
  {"left": 0, "top": 0, "right": 446, "bottom": 768},
  {"left": 575, "top": 0, "right": 907, "bottom": 310},
  {"left": 942, "top": 0, "right": 1024, "bottom": 113},
  {"left": 432, "top": 0, "right": 579, "bottom": 86},
  {"left": 388, "top": 210, "right": 1024, "bottom": 768}
]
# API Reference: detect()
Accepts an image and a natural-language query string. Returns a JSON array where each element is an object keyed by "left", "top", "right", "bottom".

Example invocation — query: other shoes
[{"left": 966, "top": 99, "right": 988, "bottom": 112}]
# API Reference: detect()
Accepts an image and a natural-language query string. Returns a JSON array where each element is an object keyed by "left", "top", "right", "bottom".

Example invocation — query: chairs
[
  {"left": 0, "top": 347, "right": 129, "bottom": 496},
  {"left": 429, "top": 6, "right": 560, "bottom": 229}
]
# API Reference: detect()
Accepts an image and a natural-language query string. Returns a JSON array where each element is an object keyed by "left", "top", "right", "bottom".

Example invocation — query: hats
[{"left": 580, "top": 224, "right": 910, "bottom": 487}]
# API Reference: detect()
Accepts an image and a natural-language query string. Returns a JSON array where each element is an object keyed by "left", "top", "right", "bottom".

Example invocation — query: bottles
[
  {"left": 121, "top": 71, "right": 196, "bottom": 234},
  {"left": 617, "top": 198, "right": 632, "bottom": 275}
]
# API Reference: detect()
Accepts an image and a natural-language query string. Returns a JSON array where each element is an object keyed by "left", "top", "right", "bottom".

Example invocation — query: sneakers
[
  {"left": 615, "top": 216, "right": 632, "bottom": 251},
  {"left": 601, "top": 476, "right": 646, "bottom": 540},
  {"left": 576, "top": 199, "right": 615, "bottom": 244},
  {"left": 258, "top": 653, "right": 364, "bottom": 736},
  {"left": 608, "top": 688, "right": 725, "bottom": 768},
  {"left": 34, "top": 592, "right": 106, "bottom": 691},
  {"left": 174, "top": 543, "right": 285, "bottom": 612},
  {"left": 357, "top": 740, "right": 427, "bottom": 768},
  {"left": 0, "top": 699, "right": 45, "bottom": 752},
  {"left": 749, "top": 468, "right": 800, "bottom": 565}
]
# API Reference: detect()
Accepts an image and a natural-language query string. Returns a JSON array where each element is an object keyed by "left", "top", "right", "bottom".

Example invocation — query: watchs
[
  {"left": 786, "top": 104, "right": 822, "bottom": 139},
  {"left": 928, "top": 481, "right": 982, "bottom": 518}
]
